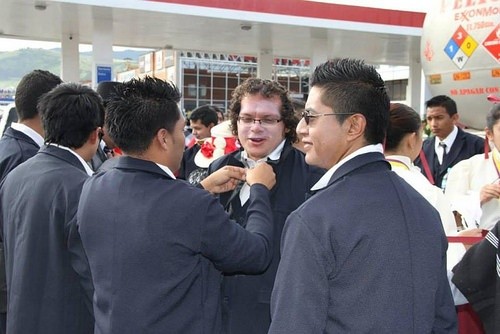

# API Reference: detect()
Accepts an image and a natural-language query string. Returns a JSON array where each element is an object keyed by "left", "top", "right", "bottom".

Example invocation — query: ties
[
  {"left": 442, "top": 144, "right": 446, "bottom": 165},
  {"left": 103, "top": 146, "right": 113, "bottom": 154}
]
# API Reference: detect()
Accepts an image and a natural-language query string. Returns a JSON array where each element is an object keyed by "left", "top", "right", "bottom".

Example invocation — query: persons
[
  {"left": 0, "top": 72, "right": 500, "bottom": 334},
  {"left": 267, "top": 57, "right": 458, "bottom": 334}
]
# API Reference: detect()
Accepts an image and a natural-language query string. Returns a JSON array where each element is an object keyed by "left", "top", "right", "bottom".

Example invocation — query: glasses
[
  {"left": 302, "top": 111, "right": 354, "bottom": 125},
  {"left": 236, "top": 116, "right": 284, "bottom": 126}
]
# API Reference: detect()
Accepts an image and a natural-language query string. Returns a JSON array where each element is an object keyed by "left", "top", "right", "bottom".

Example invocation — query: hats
[{"left": 194, "top": 119, "right": 241, "bottom": 168}]
